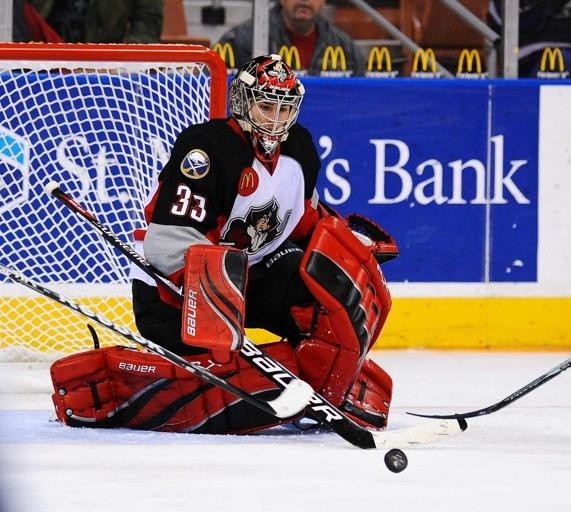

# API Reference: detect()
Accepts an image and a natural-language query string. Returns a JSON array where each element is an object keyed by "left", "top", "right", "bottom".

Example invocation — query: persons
[
  {"left": 12, "top": 2, "right": 164, "bottom": 42},
  {"left": 216, "top": 0, "right": 372, "bottom": 70},
  {"left": 49, "top": 52, "right": 401, "bottom": 438}
]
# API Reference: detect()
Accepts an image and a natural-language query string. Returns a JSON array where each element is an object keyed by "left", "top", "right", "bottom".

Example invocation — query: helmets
[{"left": 229, "top": 54, "right": 305, "bottom": 157}]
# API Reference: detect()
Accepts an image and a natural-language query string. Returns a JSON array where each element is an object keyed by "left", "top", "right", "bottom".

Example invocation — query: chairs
[{"left": 398, "top": 0, "right": 496, "bottom": 77}]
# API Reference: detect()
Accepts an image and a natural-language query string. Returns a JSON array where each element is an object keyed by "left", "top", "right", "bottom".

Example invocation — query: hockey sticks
[
  {"left": 406, "top": 357, "right": 571, "bottom": 419},
  {"left": 44, "top": 180, "right": 439, "bottom": 449},
  {"left": 1, "top": 264, "right": 468, "bottom": 442}
]
